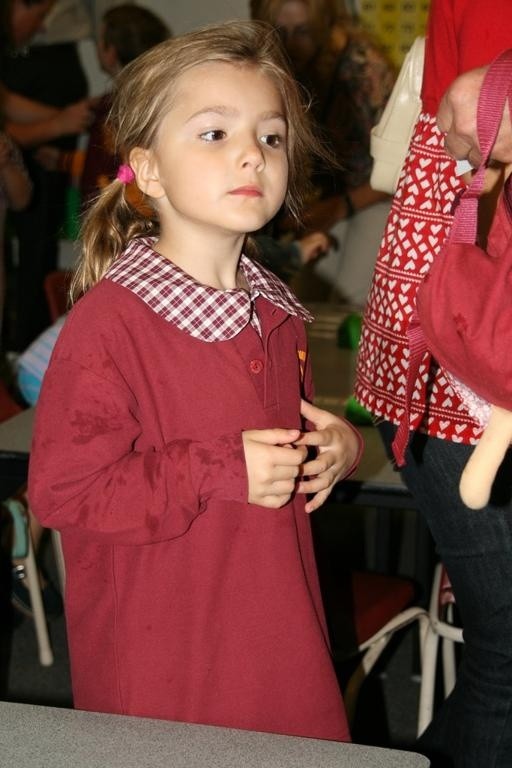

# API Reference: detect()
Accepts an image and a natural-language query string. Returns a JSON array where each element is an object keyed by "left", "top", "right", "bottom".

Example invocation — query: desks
[{"left": 0, "top": 301, "right": 434, "bottom": 768}]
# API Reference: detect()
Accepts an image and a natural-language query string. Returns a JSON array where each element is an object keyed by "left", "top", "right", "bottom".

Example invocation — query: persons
[
  {"left": 355, "top": 2, "right": 511, "bottom": 768},
  {"left": 0, "top": 0, "right": 375, "bottom": 355},
  {"left": 28, "top": 32, "right": 363, "bottom": 742}
]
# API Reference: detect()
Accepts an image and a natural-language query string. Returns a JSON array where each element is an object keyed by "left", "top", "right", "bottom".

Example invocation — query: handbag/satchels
[{"left": 418, "top": 242, "right": 512, "bottom": 411}]
